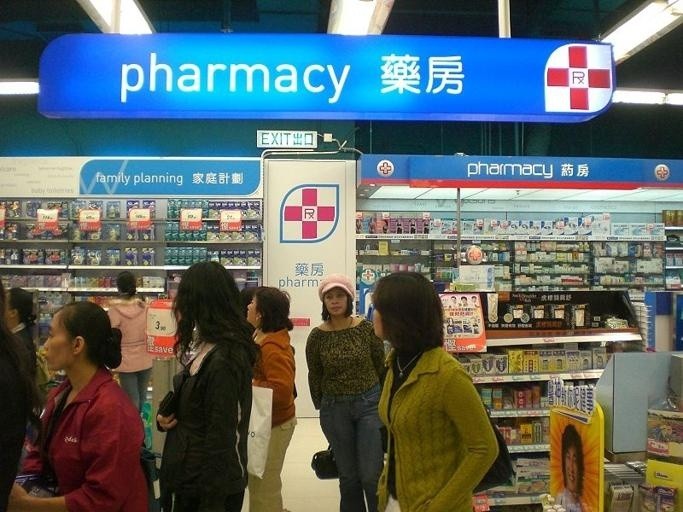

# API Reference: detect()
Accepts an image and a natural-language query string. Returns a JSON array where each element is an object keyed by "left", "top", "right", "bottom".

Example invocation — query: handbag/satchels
[
  {"left": 471, "top": 406, "right": 515, "bottom": 496},
  {"left": 139, "top": 442, "right": 164, "bottom": 511},
  {"left": 311, "top": 443, "right": 339, "bottom": 479},
  {"left": 247, "top": 384, "right": 275, "bottom": 482}
]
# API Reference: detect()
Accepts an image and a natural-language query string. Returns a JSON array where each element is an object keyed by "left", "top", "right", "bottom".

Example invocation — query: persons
[
  {"left": 306, "top": 273, "right": 390, "bottom": 512},
  {"left": 449, "top": 296, "right": 480, "bottom": 308},
  {"left": 0, "top": 284, "right": 45, "bottom": 512},
  {"left": 369, "top": 272, "right": 499, "bottom": 512},
  {"left": 154, "top": 261, "right": 264, "bottom": 512},
  {"left": 555, "top": 424, "right": 587, "bottom": 512},
  {"left": 7, "top": 302, "right": 148, "bottom": 512},
  {"left": 5, "top": 287, "right": 39, "bottom": 380},
  {"left": 107, "top": 273, "right": 153, "bottom": 414},
  {"left": 244, "top": 287, "right": 295, "bottom": 512}
]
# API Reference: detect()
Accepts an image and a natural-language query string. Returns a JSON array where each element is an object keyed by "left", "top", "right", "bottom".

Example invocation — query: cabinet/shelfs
[
  {"left": 442, "top": 333, "right": 682, "bottom": 511},
  {"left": 357, "top": 198, "right": 682, "bottom": 330},
  {"left": 0, "top": 197, "right": 263, "bottom": 400}
]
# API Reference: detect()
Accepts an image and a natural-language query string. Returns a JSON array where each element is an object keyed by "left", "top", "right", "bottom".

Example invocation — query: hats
[{"left": 317, "top": 272, "right": 356, "bottom": 303}]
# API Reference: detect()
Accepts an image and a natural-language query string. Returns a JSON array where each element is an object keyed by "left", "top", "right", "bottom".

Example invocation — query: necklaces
[
  {"left": 396, "top": 348, "right": 424, "bottom": 378},
  {"left": 328, "top": 316, "right": 353, "bottom": 331}
]
